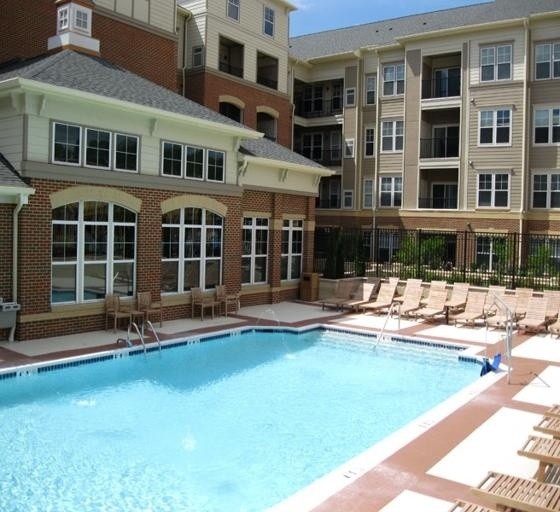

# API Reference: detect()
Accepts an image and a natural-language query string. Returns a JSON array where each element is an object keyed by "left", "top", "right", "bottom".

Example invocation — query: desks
[
  {"left": 211, "top": 300, "right": 221, "bottom": 319},
  {"left": 126, "top": 309, "right": 144, "bottom": 330}
]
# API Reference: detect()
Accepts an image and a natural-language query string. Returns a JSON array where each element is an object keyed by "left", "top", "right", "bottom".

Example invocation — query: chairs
[
  {"left": 191, "top": 287, "right": 215, "bottom": 320},
  {"left": 215, "top": 284, "right": 240, "bottom": 318},
  {"left": 322, "top": 260, "right": 559, "bottom": 337},
  {"left": 104, "top": 294, "right": 132, "bottom": 332},
  {"left": 445, "top": 401, "right": 560, "bottom": 512},
  {"left": 137, "top": 291, "right": 162, "bottom": 327}
]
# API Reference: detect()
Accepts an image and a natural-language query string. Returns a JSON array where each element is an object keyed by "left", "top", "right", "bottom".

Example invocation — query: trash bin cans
[{"left": 299, "top": 272, "right": 319, "bottom": 302}]
